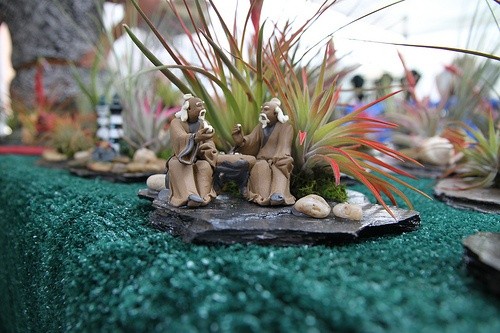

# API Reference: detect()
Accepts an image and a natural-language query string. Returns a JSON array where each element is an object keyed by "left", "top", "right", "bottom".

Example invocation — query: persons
[
  {"left": 0, "top": 0, "right": 161, "bottom": 139},
  {"left": 232, "top": 97, "right": 297, "bottom": 206},
  {"left": 328, "top": 62, "right": 472, "bottom": 126},
  {"left": 164, "top": 93, "right": 219, "bottom": 207}
]
